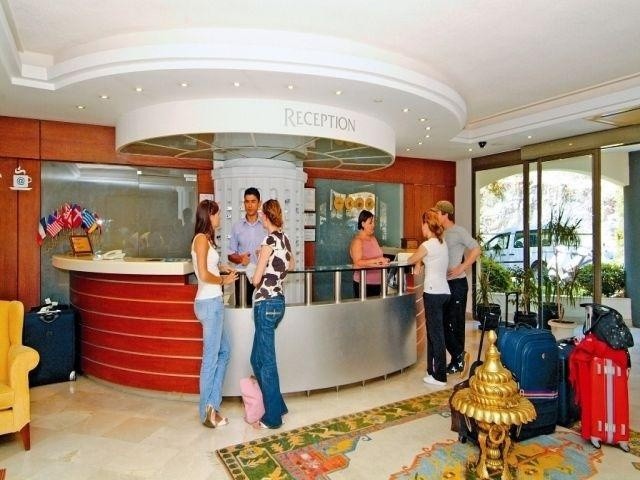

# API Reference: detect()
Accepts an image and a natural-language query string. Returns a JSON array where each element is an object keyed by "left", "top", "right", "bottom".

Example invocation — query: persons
[
  {"left": 190, "top": 199, "right": 239, "bottom": 428},
  {"left": 406, "top": 207, "right": 452, "bottom": 386},
  {"left": 248, "top": 198, "right": 295, "bottom": 431},
  {"left": 431, "top": 200, "right": 481, "bottom": 374},
  {"left": 227, "top": 188, "right": 269, "bottom": 307},
  {"left": 349, "top": 209, "right": 391, "bottom": 298}
]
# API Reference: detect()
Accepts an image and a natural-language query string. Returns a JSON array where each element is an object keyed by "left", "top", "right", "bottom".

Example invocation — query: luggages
[
  {"left": 25, "top": 305, "right": 77, "bottom": 388},
  {"left": 450, "top": 312, "right": 517, "bottom": 447},
  {"left": 558, "top": 337, "right": 580, "bottom": 429},
  {"left": 496, "top": 292, "right": 559, "bottom": 442},
  {"left": 569, "top": 303, "right": 630, "bottom": 453}
]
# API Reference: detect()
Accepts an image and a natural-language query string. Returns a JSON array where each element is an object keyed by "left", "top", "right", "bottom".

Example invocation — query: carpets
[{"left": 216, "top": 389, "right": 640, "bottom": 479}]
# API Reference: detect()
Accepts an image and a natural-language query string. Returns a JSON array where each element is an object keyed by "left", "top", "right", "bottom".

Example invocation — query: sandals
[{"left": 253, "top": 420, "right": 283, "bottom": 429}]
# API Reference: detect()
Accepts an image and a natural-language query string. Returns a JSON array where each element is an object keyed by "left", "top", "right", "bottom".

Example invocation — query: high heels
[
  {"left": 203, "top": 404, "right": 216, "bottom": 428},
  {"left": 217, "top": 413, "right": 230, "bottom": 427}
]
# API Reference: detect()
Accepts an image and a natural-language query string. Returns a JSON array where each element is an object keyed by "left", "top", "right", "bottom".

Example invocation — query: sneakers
[
  {"left": 447, "top": 364, "right": 460, "bottom": 375},
  {"left": 423, "top": 375, "right": 447, "bottom": 386},
  {"left": 457, "top": 351, "right": 470, "bottom": 379}
]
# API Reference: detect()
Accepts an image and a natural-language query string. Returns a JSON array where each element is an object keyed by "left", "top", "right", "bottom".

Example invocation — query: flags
[{"left": 36, "top": 200, "right": 104, "bottom": 247}]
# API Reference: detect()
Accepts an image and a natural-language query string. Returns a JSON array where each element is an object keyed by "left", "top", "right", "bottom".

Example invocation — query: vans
[{"left": 480, "top": 228, "right": 584, "bottom": 283}]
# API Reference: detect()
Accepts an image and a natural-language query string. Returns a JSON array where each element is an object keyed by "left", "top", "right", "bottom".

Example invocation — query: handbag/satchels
[
  {"left": 239, "top": 375, "right": 265, "bottom": 424},
  {"left": 591, "top": 306, "right": 634, "bottom": 349}
]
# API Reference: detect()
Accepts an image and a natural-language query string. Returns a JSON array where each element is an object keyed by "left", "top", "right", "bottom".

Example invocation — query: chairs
[{"left": 0, "top": 300, "right": 40, "bottom": 451}]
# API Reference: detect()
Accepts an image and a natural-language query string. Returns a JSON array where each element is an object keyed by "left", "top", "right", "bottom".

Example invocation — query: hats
[{"left": 431, "top": 201, "right": 454, "bottom": 214}]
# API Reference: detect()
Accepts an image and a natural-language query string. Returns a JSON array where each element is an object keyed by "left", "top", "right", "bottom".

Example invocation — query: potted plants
[
  {"left": 544, "top": 206, "right": 578, "bottom": 340},
  {"left": 539, "top": 267, "right": 577, "bottom": 328},
  {"left": 471, "top": 269, "right": 500, "bottom": 331},
  {"left": 505, "top": 265, "right": 538, "bottom": 328}
]
